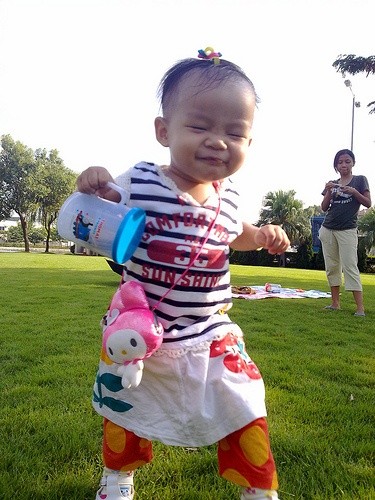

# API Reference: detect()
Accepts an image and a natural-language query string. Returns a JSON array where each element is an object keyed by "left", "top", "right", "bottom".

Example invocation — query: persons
[
  {"left": 318, "top": 149, "right": 371, "bottom": 317},
  {"left": 76, "top": 47, "right": 291, "bottom": 500}
]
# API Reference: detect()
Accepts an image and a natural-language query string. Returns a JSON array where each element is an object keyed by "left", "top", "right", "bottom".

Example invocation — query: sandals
[{"left": 95, "top": 474, "right": 135, "bottom": 500}]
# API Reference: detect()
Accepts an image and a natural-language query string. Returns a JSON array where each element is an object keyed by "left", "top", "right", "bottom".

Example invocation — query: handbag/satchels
[{"left": 100, "top": 281, "right": 163, "bottom": 389}]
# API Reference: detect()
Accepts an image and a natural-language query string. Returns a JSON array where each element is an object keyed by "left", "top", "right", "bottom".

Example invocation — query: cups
[
  {"left": 56, "top": 181, "right": 147, "bottom": 264},
  {"left": 264, "top": 283, "right": 281, "bottom": 292}
]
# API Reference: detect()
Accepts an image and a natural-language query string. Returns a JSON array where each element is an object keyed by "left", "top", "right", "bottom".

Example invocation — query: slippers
[
  {"left": 354, "top": 312, "right": 365, "bottom": 316},
  {"left": 325, "top": 304, "right": 341, "bottom": 310}
]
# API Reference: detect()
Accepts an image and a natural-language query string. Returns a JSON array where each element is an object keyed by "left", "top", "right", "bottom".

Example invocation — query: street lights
[{"left": 344, "top": 78, "right": 362, "bottom": 153}]
